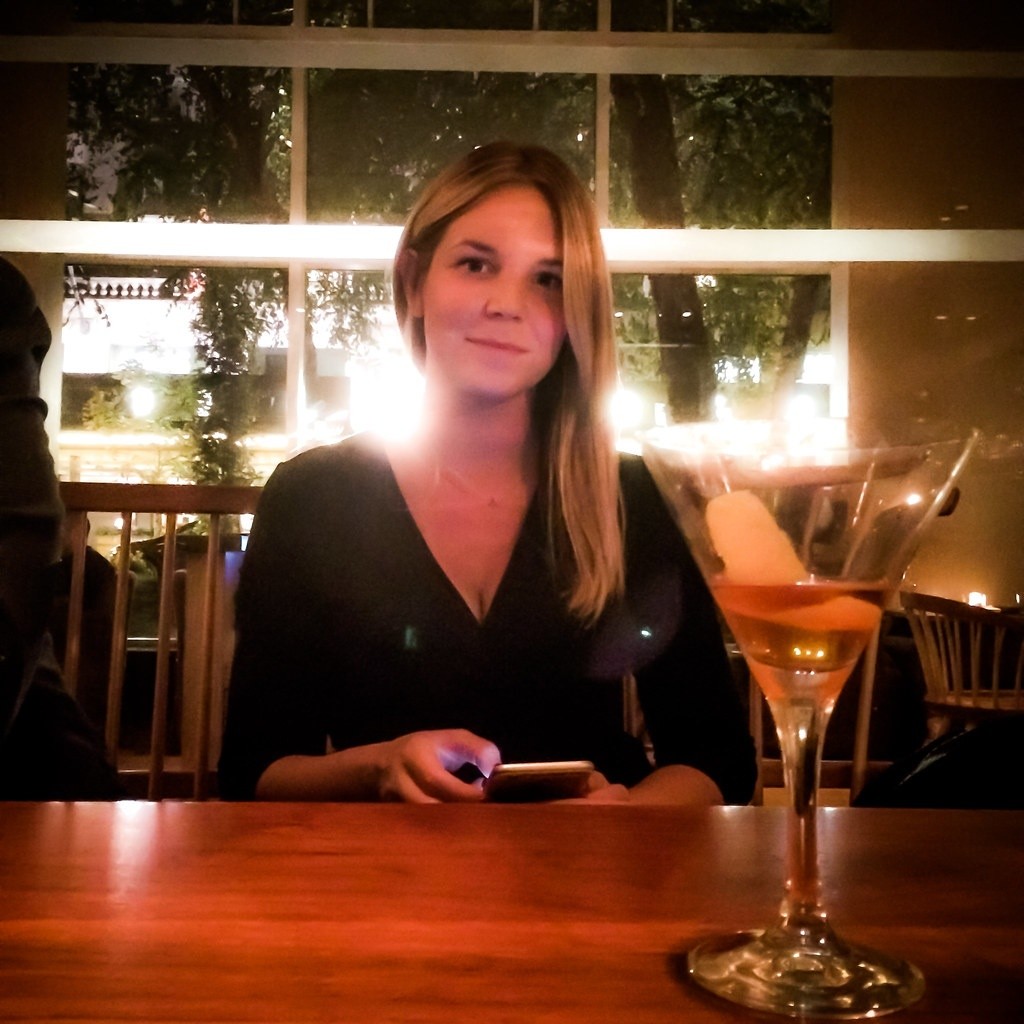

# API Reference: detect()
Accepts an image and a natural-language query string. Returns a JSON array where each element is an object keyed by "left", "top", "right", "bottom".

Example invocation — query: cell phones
[{"left": 484, "top": 761, "right": 597, "bottom": 804}]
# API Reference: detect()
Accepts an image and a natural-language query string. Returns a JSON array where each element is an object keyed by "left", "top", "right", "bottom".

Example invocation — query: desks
[{"left": 0, "top": 801, "right": 1024, "bottom": 1024}]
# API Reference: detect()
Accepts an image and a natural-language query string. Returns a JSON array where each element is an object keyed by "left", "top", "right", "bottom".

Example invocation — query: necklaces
[{"left": 414, "top": 424, "right": 537, "bottom": 510}]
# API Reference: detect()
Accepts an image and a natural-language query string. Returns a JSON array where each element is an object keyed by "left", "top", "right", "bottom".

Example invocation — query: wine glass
[{"left": 637, "top": 406, "right": 985, "bottom": 1024}]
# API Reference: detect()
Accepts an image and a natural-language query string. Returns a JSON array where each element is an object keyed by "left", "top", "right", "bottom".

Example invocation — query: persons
[
  {"left": 217, "top": 137, "right": 758, "bottom": 807},
  {"left": 1, "top": 253, "right": 111, "bottom": 802}
]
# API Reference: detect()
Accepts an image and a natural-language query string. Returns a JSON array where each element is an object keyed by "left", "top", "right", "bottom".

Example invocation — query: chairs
[
  {"left": 901, "top": 590, "right": 1024, "bottom": 749},
  {"left": 46, "top": 483, "right": 265, "bottom": 803},
  {"left": 623, "top": 455, "right": 931, "bottom": 808}
]
